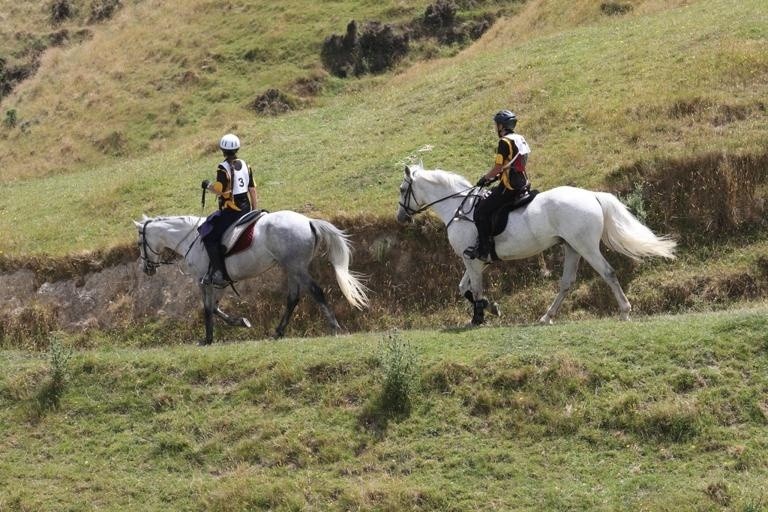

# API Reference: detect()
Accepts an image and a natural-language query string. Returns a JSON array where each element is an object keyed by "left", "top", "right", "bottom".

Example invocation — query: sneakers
[
  {"left": 201, "top": 270, "right": 224, "bottom": 285},
  {"left": 465, "top": 245, "right": 491, "bottom": 262}
]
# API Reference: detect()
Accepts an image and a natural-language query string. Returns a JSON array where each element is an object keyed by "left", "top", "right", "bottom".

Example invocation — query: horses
[
  {"left": 396, "top": 158, "right": 677, "bottom": 328},
  {"left": 131, "top": 210, "right": 374, "bottom": 347}
]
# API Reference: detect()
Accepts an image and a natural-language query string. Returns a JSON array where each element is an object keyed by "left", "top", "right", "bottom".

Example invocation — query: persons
[
  {"left": 463, "top": 109, "right": 532, "bottom": 264},
  {"left": 196, "top": 132, "right": 259, "bottom": 288}
]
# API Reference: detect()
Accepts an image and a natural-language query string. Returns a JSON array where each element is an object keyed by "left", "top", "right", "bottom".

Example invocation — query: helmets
[
  {"left": 220, "top": 134, "right": 240, "bottom": 150},
  {"left": 493, "top": 110, "right": 517, "bottom": 129}
]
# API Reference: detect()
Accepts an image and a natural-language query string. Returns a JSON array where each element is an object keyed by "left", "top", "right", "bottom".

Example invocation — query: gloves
[
  {"left": 202, "top": 180, "right": 212, "bottom": 189},
  {"left": 476, "top": 175, "right": 492, "bottom": 187}
]
